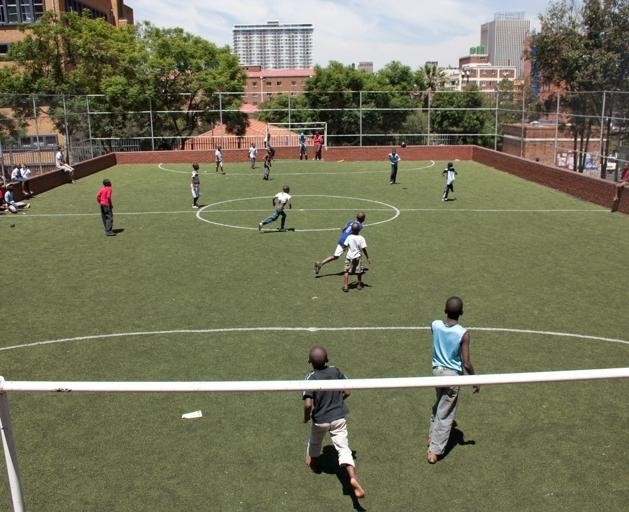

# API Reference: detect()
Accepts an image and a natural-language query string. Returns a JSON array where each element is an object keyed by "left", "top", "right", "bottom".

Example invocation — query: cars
[{"left": 554, "top": 148, "right": 617, "bottom": 172}]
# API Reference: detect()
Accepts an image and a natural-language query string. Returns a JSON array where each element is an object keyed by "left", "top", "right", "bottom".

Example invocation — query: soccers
[{"left": 401, "top": 141, "right": 406, "bottom": 148}]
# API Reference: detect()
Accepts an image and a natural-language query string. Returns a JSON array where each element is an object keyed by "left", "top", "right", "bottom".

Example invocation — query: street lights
[
  {"left": 461, "top": 70, "right": 465, "bottom": 84},
  {"left": 466, "top": 70, "right": 470, "bottom": 82}
]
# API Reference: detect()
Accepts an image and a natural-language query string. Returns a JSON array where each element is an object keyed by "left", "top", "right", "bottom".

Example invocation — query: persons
[
  {"left": 427, "top": 296, "right": 480, "bottom": 464},
  {"left": 190, "top": 163, "right": 200, "bottom": 209},
  {"left": 214, "top": 145, "right": 226, "bottom": 174},
  {"left": 302, "top": 346, "right": 365, "bottom": 499},
  {"left": 0, "top": 163, "right": 34, "bottom": 213},
  {"left": 388, "top": 148, "right": 401, "bottom": 184},
  {"left": 343, "top": 223, "right": 369, "bottom": 292},
  {"left": 96, "top": 178, "right": 116, "bottom": 236},
  {"left": 56, "top": 145, "right": 78, "bottom": 184},
  {"left": 248, "top": 131, "right": 323, "bottom": 182},
  {"left": 315, "top": 212, "right": 365, "bottom": 274},
  {"left": 442, "top": 162, "right": 458, "bottom": 200},
  {"left": 614, "top": 160, "right": 629, "bottom": 201},
  {"left": 258, "top": 184, "right": 292, "bottom": 232}
]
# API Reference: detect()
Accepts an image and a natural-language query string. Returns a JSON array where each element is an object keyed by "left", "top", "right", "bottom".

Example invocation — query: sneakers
[{"left": 314, "top": 260, "right": 321, "bottom": 274}]
